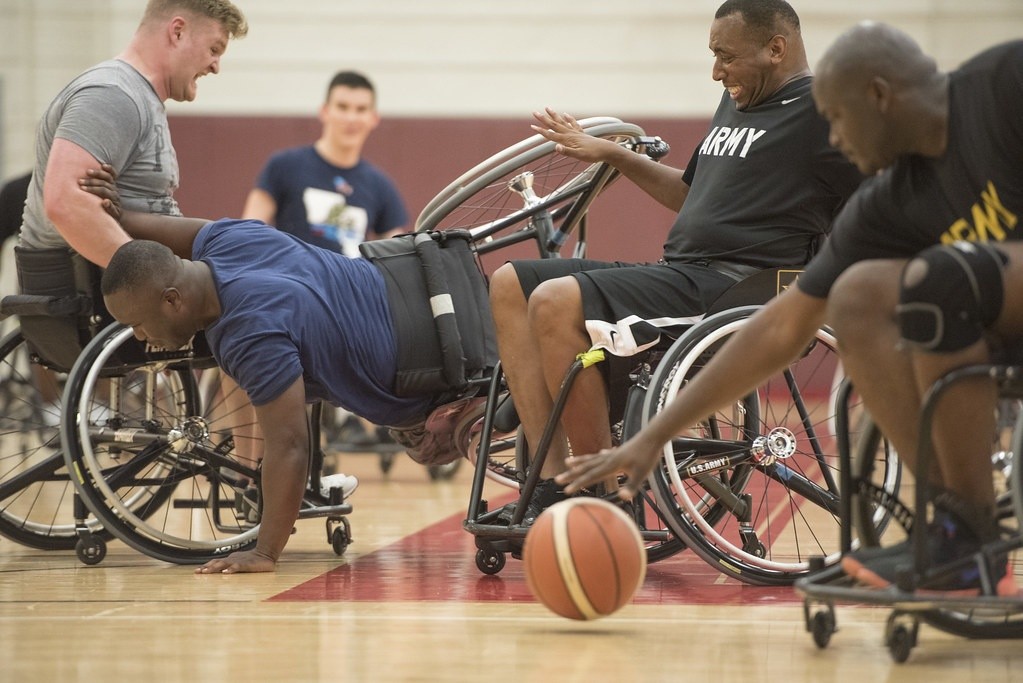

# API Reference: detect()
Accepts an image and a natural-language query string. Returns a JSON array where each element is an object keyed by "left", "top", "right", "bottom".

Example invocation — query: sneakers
[
  {"left": 234, "top": 473, "right": 358, "bottom": 527},
  {"left": 498, "top": 478, "right": 597, "bottom": 528},
  {"left": 841, "top": 502, "right": 1016, "bottom": 600}
]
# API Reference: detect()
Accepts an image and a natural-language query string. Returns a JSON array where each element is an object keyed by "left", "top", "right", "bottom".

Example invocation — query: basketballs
[{"left": 519, "top": 493, "right": 652, "bottom": 622}]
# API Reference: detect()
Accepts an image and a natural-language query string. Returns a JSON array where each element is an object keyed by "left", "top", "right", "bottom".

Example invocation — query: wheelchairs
[
  {"left": 2, "top": 117, "right": 902, "bottom": 589},
  {"left": 793, "top": 363, "right": 1023, "bottom": 663}
]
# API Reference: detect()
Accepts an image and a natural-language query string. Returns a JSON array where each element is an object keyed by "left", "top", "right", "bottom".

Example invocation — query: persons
[
  {"left": 17, "top": 0, "right": 359, "bottom": 520},
  {"left": 77, "top": 164, "right": 503, "bottom": 576},
  {"left": 554, "top": 22, "right": 1023, "bottom": 594},
  {"left": 242, "top": 70, "right": 412, "bottom": 257},
  {"left": 460, "top": 3, "right": 870, "bottom": 539}
]
